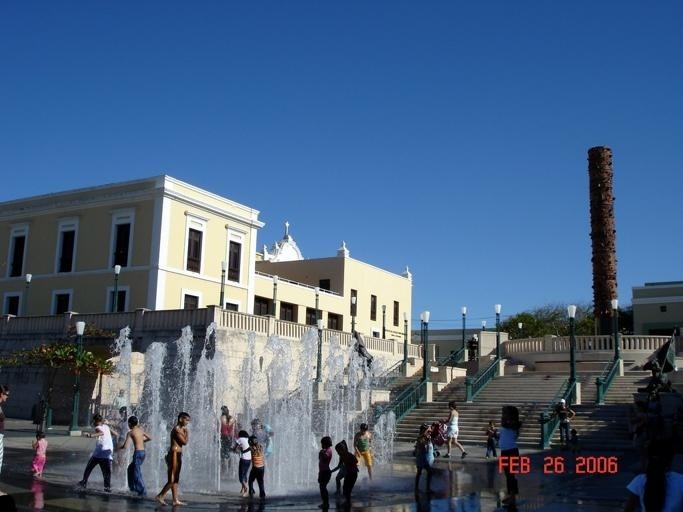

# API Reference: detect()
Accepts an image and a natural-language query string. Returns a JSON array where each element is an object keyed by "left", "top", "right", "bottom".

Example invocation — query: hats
[{"left": 558, "top": 398, "right": 566, "bottom": 404}]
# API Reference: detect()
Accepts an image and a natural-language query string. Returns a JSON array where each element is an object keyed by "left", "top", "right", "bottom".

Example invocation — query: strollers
[{"left": 413, "top": 420, "right": 445, "bottom": 460}]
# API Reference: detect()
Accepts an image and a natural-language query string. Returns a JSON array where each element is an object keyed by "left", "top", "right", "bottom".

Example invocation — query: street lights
[
  {"left": 401, "top": 298, "right": 625, "bottom": 406},
  {"left": 215, "top": 261, "right": 391, "bottom": 346},
  {"left": 68, "top": 319, "right": 87, "bottom": 438},
  {"left": 312, "top": 317, "right": 327, "bottom": 384},
  {"left": 109, "top": 261, "right": 126, "bottom": 321},
  {"left": 18, "top": 270, "right": 33, "bottom": 318}
]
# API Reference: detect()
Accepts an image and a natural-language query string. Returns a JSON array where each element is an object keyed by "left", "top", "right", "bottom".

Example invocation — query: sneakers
[
  {"left": 442, "top": 454, "right": 450, "bottom": 458},
  {"left": 461, "top": 452, "right": 468, "bottom": 459}
]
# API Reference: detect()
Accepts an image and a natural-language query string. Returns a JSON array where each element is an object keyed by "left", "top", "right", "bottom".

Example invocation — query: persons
[
  {"left": 316, "top": 437, "right": 332, "bottom": 512},
  {"left": 484, "top": 420, "right": 498, "bottom": 460},
  {"left": 497, "top": 406, "right": 523, "bottom": 505},
  {"left": 330, "top": 441, "right": 359, "bottom": 512},
  {"left": 442, "top": 401, "right": 467, "bottom": 459},
  {"left": 76, "top": 390, "right": 274, "bottom": 507},
  {"left": 29, "top": 477, "right": 44, "bottom": 511},
  {"left": 552, "top": 399, "right": 575, "bottom": 446},
  {"left": 30, "top": 430, "right": 48, "bottom": 479},
  {"left": 623, "top": 357, "right": 682, "bottom": 512},
  {"left": 0, "top": 386, "right": 9, "bottom": 473},
  {"left": 414, "top": 422, "right": 436, "bottom": 495},
  {"left": 353, "top": 422, "right": 374, "bottom": 484},
  {"left": 465, "top": 336, "right": 478, "bottom": 360}
]
